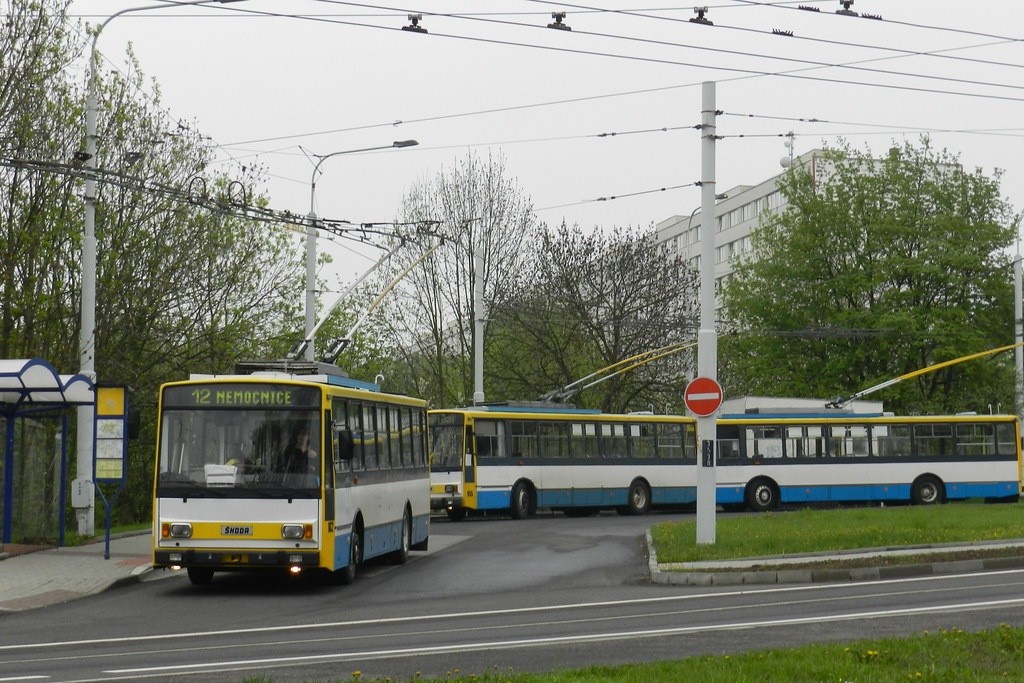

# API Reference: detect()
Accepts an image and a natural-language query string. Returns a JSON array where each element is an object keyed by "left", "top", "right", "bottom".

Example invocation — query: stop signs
[{"left": 685, "top": 376, "right": 724, "bottom": 416}]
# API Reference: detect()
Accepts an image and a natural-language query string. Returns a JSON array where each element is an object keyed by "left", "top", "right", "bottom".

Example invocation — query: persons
[{"left": 227, "top": 442, "right": 248, "bottom": 474}]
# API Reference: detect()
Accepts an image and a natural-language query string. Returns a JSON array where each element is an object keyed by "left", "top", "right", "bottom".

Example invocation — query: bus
[
  {"left": 425, "top": 398, "right": 1024, "bottom": 523},
  {"left": 152, "top": 360, "right": 436, "bottom": 588}
]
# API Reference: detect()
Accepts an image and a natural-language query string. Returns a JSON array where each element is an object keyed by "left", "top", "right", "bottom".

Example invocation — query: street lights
[{"left": 304, "top": 140, "right": 421, "bottom": 359}]
